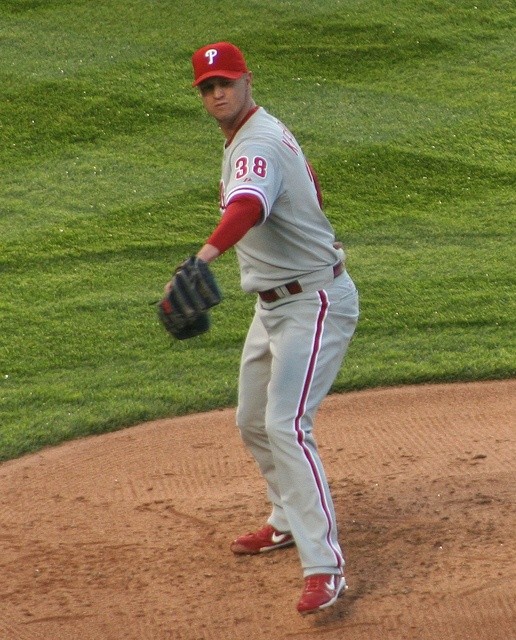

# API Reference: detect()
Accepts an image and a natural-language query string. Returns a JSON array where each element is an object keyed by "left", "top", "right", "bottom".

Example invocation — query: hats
[{"left": 192, "top": 42, "right": 248, "bottom": 87}]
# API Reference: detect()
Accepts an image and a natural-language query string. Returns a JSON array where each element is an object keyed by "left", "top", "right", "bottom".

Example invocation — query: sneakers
[
  {"left": 230, "top": 524, "right": 295, "bottom": 554},
  {"left": 296, "top": 574, "right": 346, "bottom": 615}
]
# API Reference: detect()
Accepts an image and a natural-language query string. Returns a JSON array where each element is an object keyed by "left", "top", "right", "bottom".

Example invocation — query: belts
[{"left": 257, "top": 259, "right": 344, "bottom": 303}]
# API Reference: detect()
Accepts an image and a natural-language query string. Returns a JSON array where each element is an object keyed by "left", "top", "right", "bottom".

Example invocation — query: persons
[{"left": 155, "top": 39, "right": 361, "bottom": 618}]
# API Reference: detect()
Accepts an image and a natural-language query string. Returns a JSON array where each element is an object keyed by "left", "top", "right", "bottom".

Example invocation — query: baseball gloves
[{"left": 155, "top": 256, "right": 222, "bottom": 340}]
